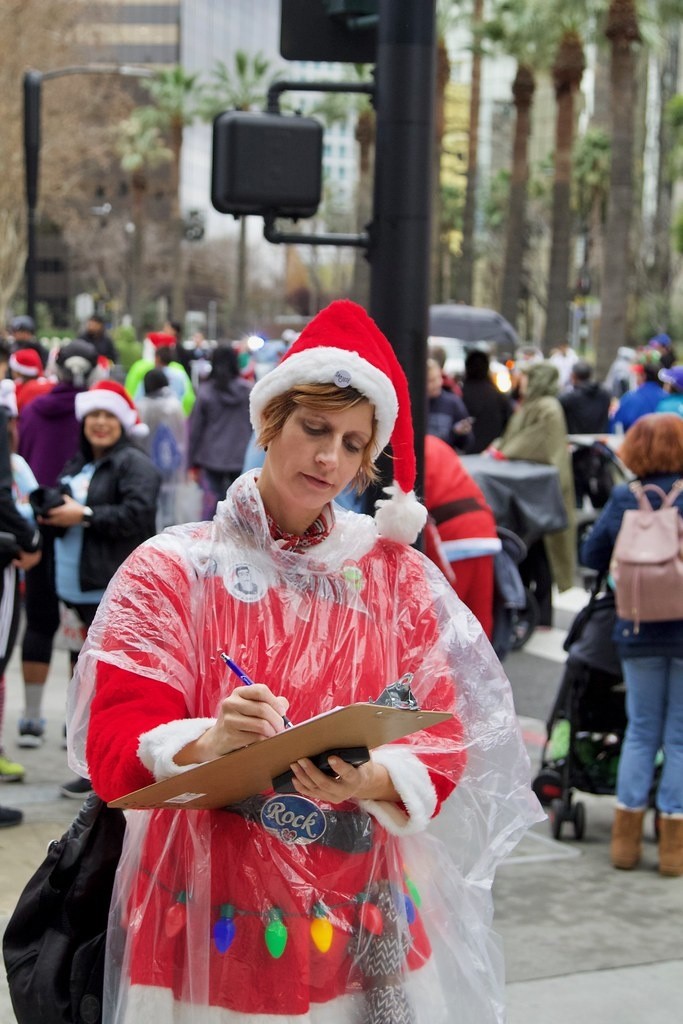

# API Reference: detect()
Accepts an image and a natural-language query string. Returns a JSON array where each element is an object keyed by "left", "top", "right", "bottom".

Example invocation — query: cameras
[{"left": 28, "top": 482, "right": 74, "bottom": 516}]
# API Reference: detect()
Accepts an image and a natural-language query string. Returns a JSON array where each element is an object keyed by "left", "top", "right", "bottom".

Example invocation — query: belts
[
  {"left": 225, "top": 790, "right": 372, "bottom": 856},
  {"left": 428, "top": 497, "right": 482, "bottom": 527}
]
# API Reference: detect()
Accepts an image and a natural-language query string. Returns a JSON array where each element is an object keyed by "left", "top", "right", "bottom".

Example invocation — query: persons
[
  {"left": 0, "top": 316, "right": 683, "bottom": 827},
  {"left": 87, "top": 300, "right": 473, "bottom": 1024},
  {"left": 602, "top": 412, "right": 683, "bottom": 881}
]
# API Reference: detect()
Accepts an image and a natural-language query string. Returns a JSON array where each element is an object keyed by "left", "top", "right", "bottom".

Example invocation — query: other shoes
[
  {"left": 0, "top": 754, "right": 26, "bottom": 781},
  {"left": 16, "top": 717, "right": 46, "bottom": 746},
  {"left": 0, "top": 806, "right": 22, "bottom": 828},
  {"left": 59, "top": 775, "right": 92, "bottom": 800}
]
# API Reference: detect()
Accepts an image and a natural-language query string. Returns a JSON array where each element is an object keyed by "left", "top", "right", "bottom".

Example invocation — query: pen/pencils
[{"left": 220, "top": 655, "right": 299, "bottom": 726}]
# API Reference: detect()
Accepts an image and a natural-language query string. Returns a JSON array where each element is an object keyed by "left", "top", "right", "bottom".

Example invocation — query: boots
[
  {"left": 610, "top": 805, "right": 649, "bottom": 870},
  {"left": 655, "top": 812, "right": 683, "bottom": 877}
]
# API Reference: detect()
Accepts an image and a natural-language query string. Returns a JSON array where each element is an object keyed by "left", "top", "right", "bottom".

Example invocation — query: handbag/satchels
[{"left": 3, "top": 791, "right": 126, "bottom": 1024}]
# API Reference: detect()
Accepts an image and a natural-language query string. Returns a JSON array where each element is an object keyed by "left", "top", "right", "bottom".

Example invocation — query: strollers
[{"left": 528, "top": 567, "right": 663, "bottom": 845}]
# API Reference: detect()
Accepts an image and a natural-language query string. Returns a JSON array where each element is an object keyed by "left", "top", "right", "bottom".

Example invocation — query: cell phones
[{"left": 272, "top": 748, "right": 372, "bottom": 794}]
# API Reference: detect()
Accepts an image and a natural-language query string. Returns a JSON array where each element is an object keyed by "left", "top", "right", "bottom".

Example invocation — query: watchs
[{"left": 82, "top": 506, "right": 93, "bottom": 528}]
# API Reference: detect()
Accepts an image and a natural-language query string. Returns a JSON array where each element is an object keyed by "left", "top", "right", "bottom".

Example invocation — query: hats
[
  {"left": 248, "top": 300, "right": 427, "bottom": 547},
  {"left": 74, "top": 379, "right": 150, "bottom": 441},
  {"left": 143, "top": 332, "right": 176, "bottom": 364},
  {"left": 633, "top": 361, "right": 663, "bottom": 387},
  {"left": 9, "top": 349, "right": 48, "bottom": 384},
  {"left": 657, "top": 363, "right": 683, "bottom": 393}
]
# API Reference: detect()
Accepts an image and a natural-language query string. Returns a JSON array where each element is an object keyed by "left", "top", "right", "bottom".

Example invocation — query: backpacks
[{"left": 609, "top": 481, "right": 683, "bottom": 621}]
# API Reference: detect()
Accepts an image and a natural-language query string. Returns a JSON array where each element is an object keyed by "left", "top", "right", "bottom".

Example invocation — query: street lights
[{"left": 19, "top": 61, "right": 156, "bottom": 321}]
